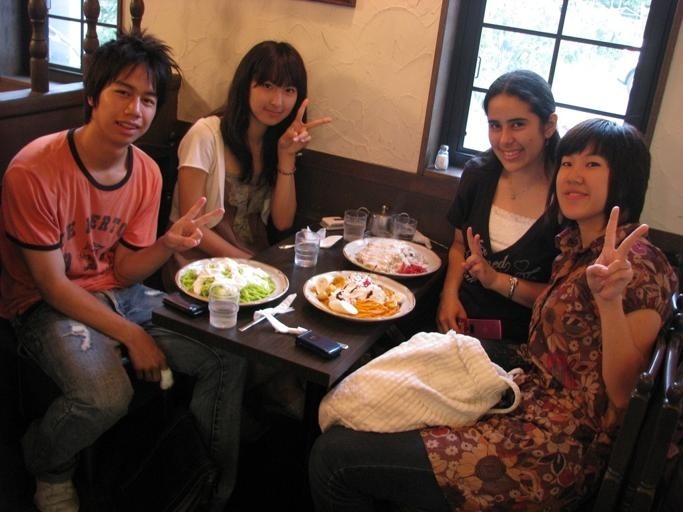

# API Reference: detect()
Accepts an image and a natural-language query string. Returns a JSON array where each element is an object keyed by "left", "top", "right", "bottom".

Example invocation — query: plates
[
  {"left": 172, "top": 256, "right": 291, "bottom": 308},
  {"left": 301, "top": 267, "right": 417, "bottom": 325},
  {"left": 345, "top": 237, "right": 443, "bottom": 279}
]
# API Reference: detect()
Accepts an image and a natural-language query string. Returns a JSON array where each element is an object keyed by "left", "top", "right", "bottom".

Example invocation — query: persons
[
  {"left": 433, "top": 68, "right": 564, "bottom": 352},
  {"left": 0, "top": 26, "right": 243, "bottom": 508},
  {"left": 163, "top": 39, "right": 334, "bottom": 427},
  {"left": 306, "top": 116, "right": 681, "bottom": 508}
]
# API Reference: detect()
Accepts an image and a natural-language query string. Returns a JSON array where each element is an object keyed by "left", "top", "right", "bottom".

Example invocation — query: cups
[
  {"left": 390, "top": 212, "right": 418, "bottom": 242},
  {"left": 342, "top": 209, "right": 366, "bottom": 242},
  {"left": 293, "top": 232, "right": 321, "bottom": 268},
  {"left": 370, "top": 204, "right": 394, "bottom": 238},
  {"left": 206, "top": 287, "right": 238, "bottom": 330}
]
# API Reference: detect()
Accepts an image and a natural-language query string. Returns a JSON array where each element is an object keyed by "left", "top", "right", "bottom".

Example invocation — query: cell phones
[
  {"left": 296, "top": 331, "right": 341, "bottom": 360},
  {"left": 456, "top": 318, "right": 502, "bottom": 340},
  {"left": 320, "top": 216, "right": 344, "bottom": 231},
  {"left": 164, "top": 292, "right": 207, "bottom": 318}
]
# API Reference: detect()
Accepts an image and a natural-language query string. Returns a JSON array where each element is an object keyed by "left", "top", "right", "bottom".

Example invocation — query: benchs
[{"left": 1, "top": 77, "right": 179, "bottom": 376}]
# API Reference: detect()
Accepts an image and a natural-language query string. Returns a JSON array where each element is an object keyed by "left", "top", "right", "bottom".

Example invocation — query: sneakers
[{"left": 33, "top": 478, "right": 80, "bottom": 512}]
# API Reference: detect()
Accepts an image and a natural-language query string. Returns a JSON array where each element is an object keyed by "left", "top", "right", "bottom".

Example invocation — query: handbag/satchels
[{"left": 317, "top": 329, "right": 524, "bottom": 434}]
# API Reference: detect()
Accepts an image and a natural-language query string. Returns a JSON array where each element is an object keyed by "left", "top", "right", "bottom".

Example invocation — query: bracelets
[
  {"left": 275, "top": 166, "right": 297, "bottom": 177},
  {"left": 506, "top": 275, "right": 518, "bottom": 299}
]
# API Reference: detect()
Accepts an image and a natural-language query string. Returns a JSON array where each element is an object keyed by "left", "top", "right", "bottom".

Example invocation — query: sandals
[{"left": 271, "top": 384, "right": 305, "bottom": 420}]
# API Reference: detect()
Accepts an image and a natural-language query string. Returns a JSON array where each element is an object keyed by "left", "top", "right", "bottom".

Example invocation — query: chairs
[{"left": 602, "top": 250, "right": 683, "bottom": 512}]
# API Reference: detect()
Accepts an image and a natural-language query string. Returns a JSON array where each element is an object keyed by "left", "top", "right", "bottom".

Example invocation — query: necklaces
[{"left": 500, "top": 176, "right": 546, "bottom": 200}]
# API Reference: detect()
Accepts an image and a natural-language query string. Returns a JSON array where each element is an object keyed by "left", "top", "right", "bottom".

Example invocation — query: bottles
[{"left": 433, "top": 143, "right": 453, "bottom": 173}]
